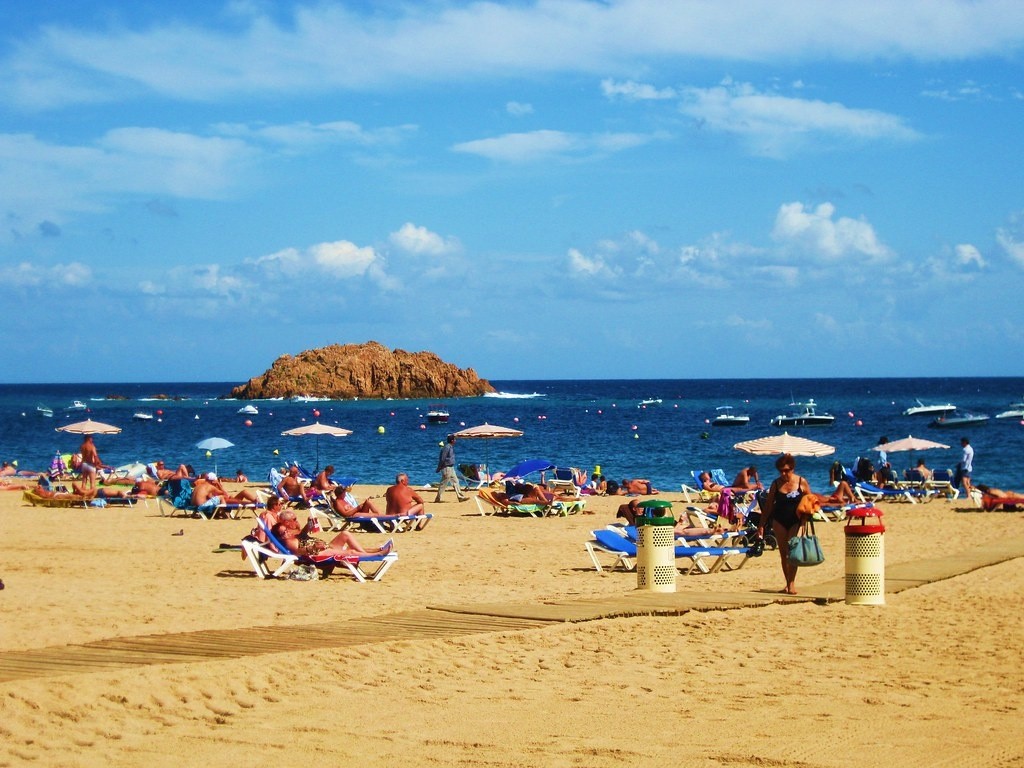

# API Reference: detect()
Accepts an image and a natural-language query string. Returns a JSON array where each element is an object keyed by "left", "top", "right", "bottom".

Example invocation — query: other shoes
[
  {"left": 435, "top": 499, "right": 446, "bottom": 503},
  {"left": 459, "top": 496, "right": 470, "bottom": 502}
]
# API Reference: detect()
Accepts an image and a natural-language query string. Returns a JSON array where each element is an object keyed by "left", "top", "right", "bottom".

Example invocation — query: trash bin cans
[
  {"left": 844, "top": 506, "right": 886, "bottom": 605},
  {"left": 635, "top": 499, "right": 677, "bottom": 593}
]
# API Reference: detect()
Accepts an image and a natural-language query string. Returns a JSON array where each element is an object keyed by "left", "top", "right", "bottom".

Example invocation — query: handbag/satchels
[
  {"left": 289, "top": 565, "right": 320, "bottom": 583},
  {"left": 788, "top": 514, "right": 824, "bottom": 566}
]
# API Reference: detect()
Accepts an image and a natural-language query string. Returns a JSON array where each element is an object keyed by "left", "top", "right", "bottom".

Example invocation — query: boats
[
  {"left": 427, "top": 404, "right": 449, "bottom": 423},
  {"left": 36, "top": 405, "right": 53, "bottom": 417},
  {"left": 927, "top": 412, "right": 989, "bottom": 429},
  {"left": 237, "top": 404, "right": 259, "bottom": 415},
  {"left": 771, "top": 400, "right": 835, "bottom": 427},
  {"left": 902, "top": 398, "right": 957, "bottom": 416},
  {"left": 996, "top": 402, "right": 1024, "bottom": 425},
  {"left": 639, "top": 397, "right": 662, "bottom": 406},
  {"left": 63, "top": 401, "right": 87, "bottom": 411},
  {"left": 133, "top": 413, "right": 152, "bottom": 420},
  {"left": 711, "top": 405, "right": 750, "bottom": 425}
]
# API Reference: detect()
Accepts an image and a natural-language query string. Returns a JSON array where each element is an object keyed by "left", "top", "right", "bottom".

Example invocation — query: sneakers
[{"left": 379, "top": 538, "right": 393, "bottom": 556}]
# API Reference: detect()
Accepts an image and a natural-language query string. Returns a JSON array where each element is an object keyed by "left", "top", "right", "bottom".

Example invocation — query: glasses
[{"left": 779, "top": 469, "right": 791, "bottom": 472}]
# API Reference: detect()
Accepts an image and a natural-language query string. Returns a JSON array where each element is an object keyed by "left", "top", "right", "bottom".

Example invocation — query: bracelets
[{"left": 756, "top": 480, "right": 758, "bottom": 483}]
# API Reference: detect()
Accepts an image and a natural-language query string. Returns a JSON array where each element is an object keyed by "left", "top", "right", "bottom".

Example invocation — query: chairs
[
  {"left": 586, "top": 458, "right": 1024, "bottom": 576},
  {"left": 21, "top": 460, "right": 433, "bottom": 582},
  {"left": 456, "top": 463, "right": 592, "bottom": 518}
]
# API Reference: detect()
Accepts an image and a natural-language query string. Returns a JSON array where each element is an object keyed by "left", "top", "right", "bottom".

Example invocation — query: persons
[
  {"left": 877, "top": 463, "right": 897, "bottom": 500},
  {"left": 756, "top": 454, "right": 811, "bottom": 594},
  {"left": 0, "top": 461, "right": 37, "bottom": 476},
  {"left": 71, "top": 453, "right": 336, "bottom": 518},
  {"left": 470, "top": 463, "right": 659, "bottom": 506},
  {"left": 0, "top": 482, "right": 134, "bottom": 498},
  {"left": 911, "top": 458, "right": 931, "bottom": 502},
  {"left": 878, "top": 436, "right": 887, "bottom": 469},
  {"left": 628, "top": 462, "right": 866, "bottom": 538},
  {"left": 334, "top": 486, "right": 400, "bottom": 518},
  {"left": 80, "top": 435, "right": 97, "bottom": 489},
  {"left": 977, "top": 485, "right": 1024, "bottom": 499},
  {"left": 958, "top": 437, "right": 974, "bottom": 500},
  {"left": 434, "top": 434, "right": 470, "bottom": 503},
  {"left": 386, "top": 473, "right": 425, "bottom": 531},
  {"left": 257, "top": 496, "right": 394, "bottom": 557}
]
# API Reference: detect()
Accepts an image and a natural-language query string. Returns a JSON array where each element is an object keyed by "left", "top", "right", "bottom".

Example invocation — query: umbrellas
[
  {"left": 281, "top": 421, "right": 353, "bottom": 470},
  {"left": 54, "top": 418, "right": 122, "bottom": 434},
  {"left": 447, "top": 421, "right": 524, "bottom": 488},
  {"left": 872, "top": 435, "right": 951, "bottom": 469},
  {"left": 733, "top": 431, "right": 835, "bottom": 457},
  {"left": 196, "top": 437, "right": 235, "bottom": 476},
  {"left": 47, "top": 450, "right": 68, "bottom": 482},
  {"left": 500, "top": 459, "right": 557, "bottom": 482}
]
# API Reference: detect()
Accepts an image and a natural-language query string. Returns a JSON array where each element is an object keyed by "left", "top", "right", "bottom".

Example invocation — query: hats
[{"left": 207, "top": 472, "right": 217, "bottom": 481}]
